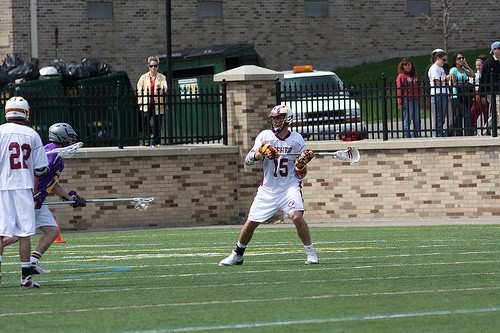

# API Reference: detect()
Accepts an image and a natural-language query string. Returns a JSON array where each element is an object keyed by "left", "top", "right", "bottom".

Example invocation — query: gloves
[
  {"left": 260, "top": 141, "right": 279, "bottom": 160},
  {"left": 295, "top": 148, "right": 315, "bottom": 170},
  {"left": 33, "top": 192, "right": 43, "bottom": 209},
  {"left": 67, "top": 190, "right": 86, "bottom": 208}
]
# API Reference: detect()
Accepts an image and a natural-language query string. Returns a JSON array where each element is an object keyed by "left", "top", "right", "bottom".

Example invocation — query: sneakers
[
  {"left": 21, "top": 276, "right": 40, "bottom": 289},
  {"left": 30, "top": 264, "right": 51, "bottom": 275},
  {"left": 305, "top": 252, "right": 320, "bottom": 265},
  {"left": 218, "top": 250, "right": 244, "bottom": 266}
]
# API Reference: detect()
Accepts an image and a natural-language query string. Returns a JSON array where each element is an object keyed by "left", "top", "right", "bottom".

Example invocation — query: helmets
[
  {"left": 5, "top": 96, "right": 30, "bottom": 122},
  {"left": 49, "top": 123, "right": 78, "bottom": 145},
  {"left": 269, "top": 105, "right": 293, "bottom": 136}
]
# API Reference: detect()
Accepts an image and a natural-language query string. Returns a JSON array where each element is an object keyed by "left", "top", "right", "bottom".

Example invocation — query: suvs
[{"left": 279, "top": 65, "right": 366, "bottom": 140}]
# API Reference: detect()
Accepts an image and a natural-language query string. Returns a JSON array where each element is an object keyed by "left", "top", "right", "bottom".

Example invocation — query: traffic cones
[{"left": 53, "top": 220, "right": 65, "bottom": 242}]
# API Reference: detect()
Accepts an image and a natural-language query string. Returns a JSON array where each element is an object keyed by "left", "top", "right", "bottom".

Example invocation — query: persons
[
  {"left": 0, "top": 96, "right": 49, "bottom": 289},
  {"left": 134, "top": 55, "right": 167, "bottom": 147},
  {"left": 395, "top": 41, "right": 500, "bottom": 137},
  {"left": 2, "top": 122, "right": 87, "bottom": 274},
  {"left": 217, "top": 105, "right": 321, "bottom": 267}
]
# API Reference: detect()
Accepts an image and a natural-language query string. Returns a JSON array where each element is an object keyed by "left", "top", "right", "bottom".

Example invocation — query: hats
[{"left": 489, "top": 41, "right": 500, "bottom": 54}]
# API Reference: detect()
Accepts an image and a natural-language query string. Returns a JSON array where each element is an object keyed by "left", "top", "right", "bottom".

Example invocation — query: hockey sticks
[
  {"left": 46, "top": 142, "right": 83, "bottom": 159},
  {"left": 41, "top": 197, "right": 154, "bottom": 213},
  {"left": 261, "top": 146, "right": 361, "bottom": 163}
]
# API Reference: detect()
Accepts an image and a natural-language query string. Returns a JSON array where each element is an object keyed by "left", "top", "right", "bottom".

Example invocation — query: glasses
[
  {"left": 457, "top": 57, "right": 464, "bottom": 60},
  {"left": 149, "top": 65, "right": 158, "bottom": 68},
  {"left": 442, "top": 59, "right": 446, "bottom": 62}
]
